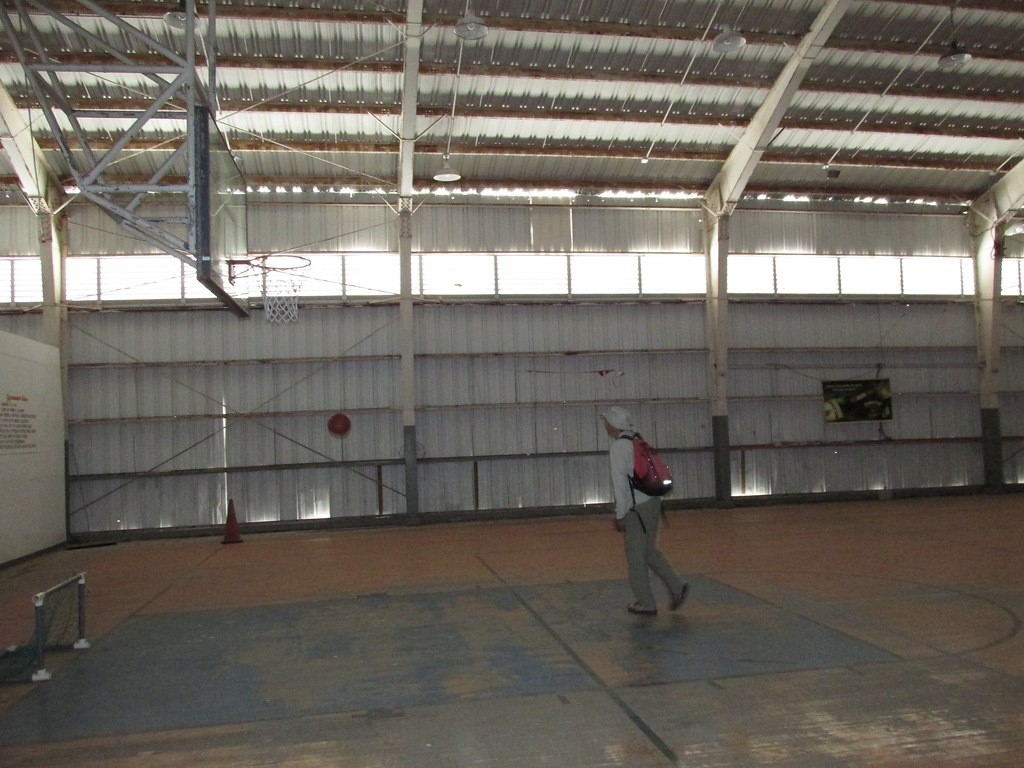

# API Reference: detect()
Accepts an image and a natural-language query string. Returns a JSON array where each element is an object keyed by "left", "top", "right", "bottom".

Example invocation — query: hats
[{"left": 600, "top": 406, "right": 631, "bottom": 431}]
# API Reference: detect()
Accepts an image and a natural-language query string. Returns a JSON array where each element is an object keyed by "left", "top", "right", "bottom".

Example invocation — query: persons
[{"left": 601, "top": 406, "right": 689, "bottom": 614}]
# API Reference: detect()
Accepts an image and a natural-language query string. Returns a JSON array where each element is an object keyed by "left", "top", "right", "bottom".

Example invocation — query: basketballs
[{"left": 326, "top": 413, "right": 351, "bottom": 434}]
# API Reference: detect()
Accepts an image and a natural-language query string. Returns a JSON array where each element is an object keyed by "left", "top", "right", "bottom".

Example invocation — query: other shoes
[
  {"left": 669, "top": 581, "right": 689, "bottom": 611},
  {"left": 627, "top": 600, "right": 658, "bottom": 615}
]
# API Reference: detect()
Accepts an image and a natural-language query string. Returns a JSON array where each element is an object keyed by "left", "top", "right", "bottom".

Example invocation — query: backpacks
[{"left": 617, "top": 432, "right": 673, "bottom": 495}]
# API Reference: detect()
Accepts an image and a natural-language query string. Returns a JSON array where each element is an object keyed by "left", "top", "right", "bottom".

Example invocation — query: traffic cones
[{"left": 220, "top": 498, "right": 244, "bottom": 544}]
